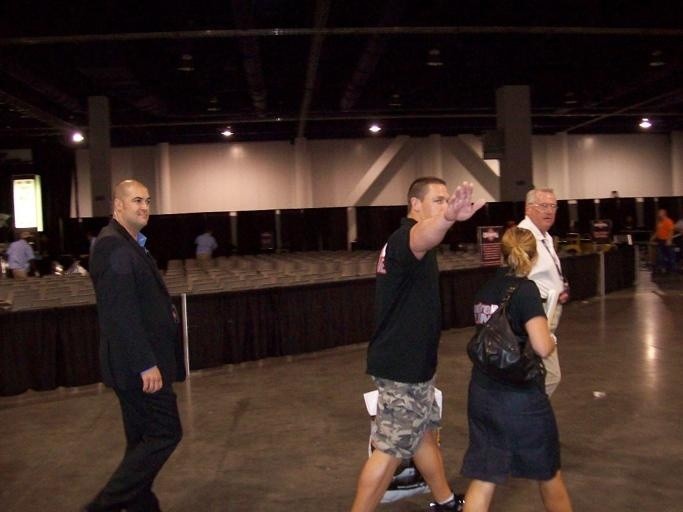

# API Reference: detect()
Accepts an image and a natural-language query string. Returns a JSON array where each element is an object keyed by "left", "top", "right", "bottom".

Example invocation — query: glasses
[{"left": 527, "top": 201, "right": 561, "bottom": 212}]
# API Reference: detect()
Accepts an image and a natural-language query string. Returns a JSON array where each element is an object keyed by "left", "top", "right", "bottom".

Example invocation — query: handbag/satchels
[{"left": 466, "top": 279, "right": 547, "bottom": 386}]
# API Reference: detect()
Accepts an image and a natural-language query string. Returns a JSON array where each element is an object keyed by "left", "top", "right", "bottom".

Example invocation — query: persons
[
  {"left": 453, "top": 225, "right": 578, "bottom": 512},
  {"left": 85, "top": 231, "right": 99, "bottom": 263},
  {"left": 80, "top": 177, "right": 188, "bottom": 510},
  {"left": 60, "top": 252, "right": 89, "bottom": 275},
  {"left": 512, "top": 186, "right": 572, "bottom": 407},
  {"left": 348, "top": 172, "right": 487, "bottom": 511},
  {"left": 650, "top": 209, "right": 675, "bottom": 268},
  {"left": 194, "top": 226, "right": 218, "bottom": 259},
  {"left": 0, "top": 231, "right": 41, "bottom": 280}
]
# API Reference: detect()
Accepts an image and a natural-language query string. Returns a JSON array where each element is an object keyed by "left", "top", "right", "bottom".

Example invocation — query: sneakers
[{"left": 425, "top": 489, "right": 465, "bottom": 512}]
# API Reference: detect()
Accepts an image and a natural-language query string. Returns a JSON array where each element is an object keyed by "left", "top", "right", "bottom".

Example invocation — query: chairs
[
  {"left": 552, "top": 241, "right": 610, "bottom": 257},
  {"left": 0, "top": 275, "right": 99, "bottom": 307},
  {"left": 158, "top": 251, "right": 386, "bottom": 295},
  {"left": 434, "top": 241, "right": 509, "bottom": 271}
]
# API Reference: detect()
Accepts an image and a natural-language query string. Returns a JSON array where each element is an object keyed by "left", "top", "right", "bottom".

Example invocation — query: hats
[{"left": 19, "top": 230, "right": 34, "bottom": 240}]
